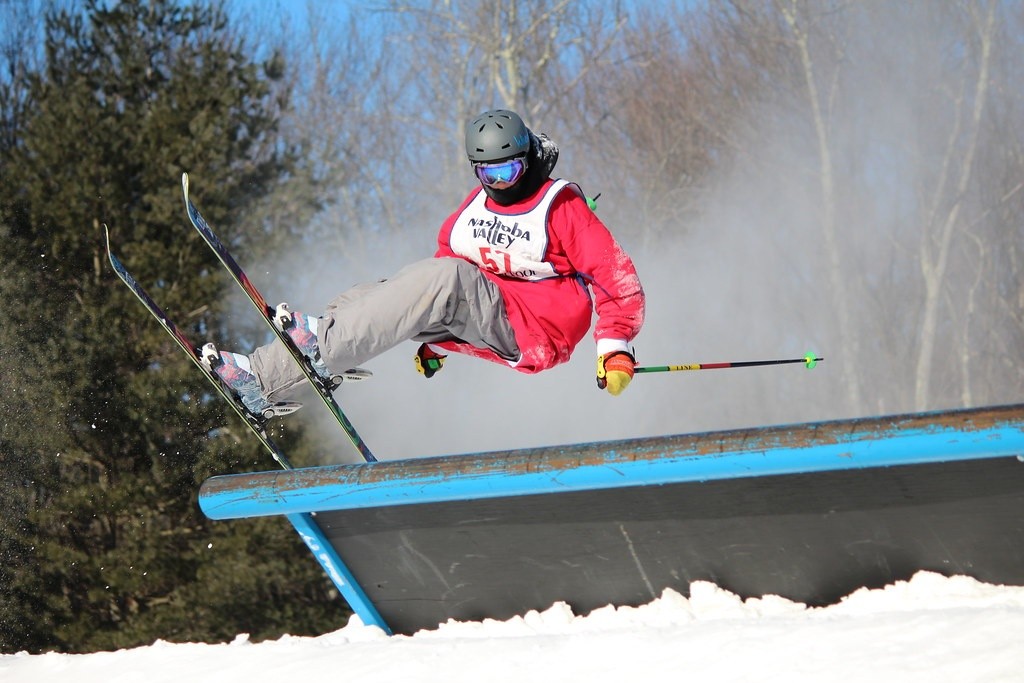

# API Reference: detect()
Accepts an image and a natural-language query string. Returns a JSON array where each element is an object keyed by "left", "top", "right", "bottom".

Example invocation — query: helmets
[{"left": 466, "top": 110, "right": 530, "bottom": 166}]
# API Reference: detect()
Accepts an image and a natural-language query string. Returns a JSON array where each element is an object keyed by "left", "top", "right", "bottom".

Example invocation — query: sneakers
[
  {"left": 212, "top": 351, "right": 278, "bottom": 414},
  {"left": 285, "top": 312, "right": 334, "bottom": 380}
]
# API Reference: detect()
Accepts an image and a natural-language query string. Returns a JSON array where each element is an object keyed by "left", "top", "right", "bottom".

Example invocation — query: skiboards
[{"left": 98, "top": 173, "right": 387, "bottom": 471}]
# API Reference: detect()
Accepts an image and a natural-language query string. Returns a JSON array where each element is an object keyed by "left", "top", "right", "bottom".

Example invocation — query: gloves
[
  {"left": 414, "top": 342, "right": 448, "bottom": 378},
  {"left": 597, "top": 349, "right": 634, "bottom": 395}
]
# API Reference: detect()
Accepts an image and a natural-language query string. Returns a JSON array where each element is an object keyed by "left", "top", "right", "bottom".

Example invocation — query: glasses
[{"left": 473, "top": 158, "right": 528, "bottom": 186}]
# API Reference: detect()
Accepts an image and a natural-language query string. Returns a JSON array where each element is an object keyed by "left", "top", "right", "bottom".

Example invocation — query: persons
[{"left": 196, "top": 110, "right": 644, "bottom": 429}]
